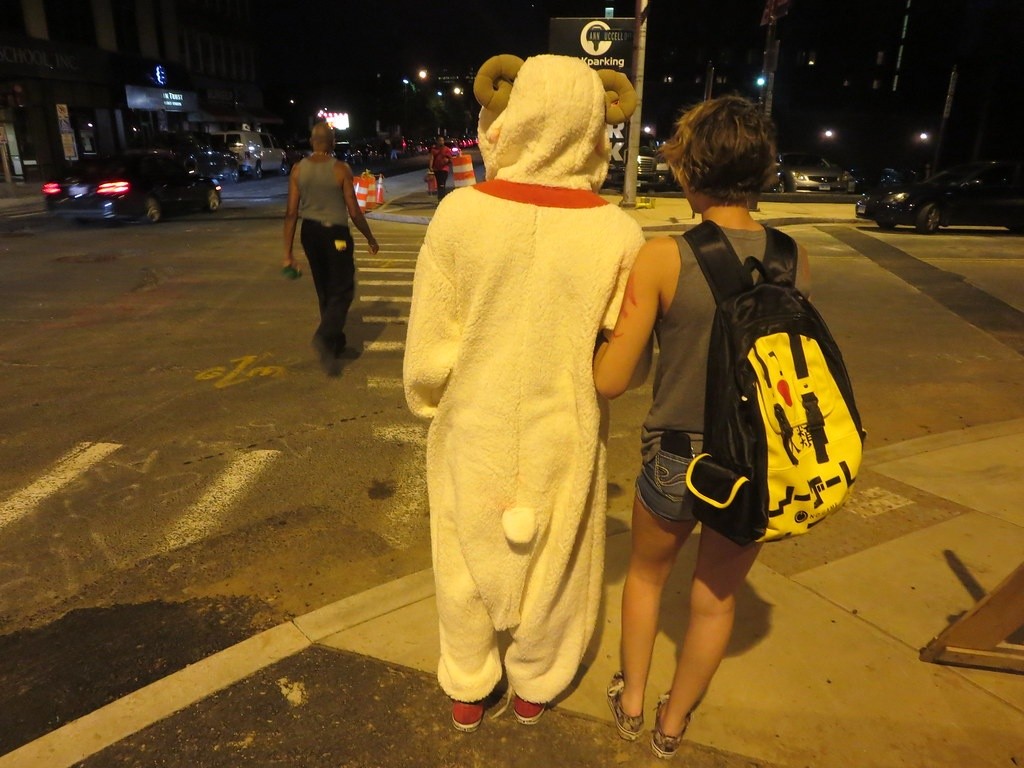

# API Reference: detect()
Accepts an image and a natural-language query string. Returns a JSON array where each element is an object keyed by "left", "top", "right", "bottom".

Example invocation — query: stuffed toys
[{"left": 403, "top": 55, "right": 654, "bottom": 732}]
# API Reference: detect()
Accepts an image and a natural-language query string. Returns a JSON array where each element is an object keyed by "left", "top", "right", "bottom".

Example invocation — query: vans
[{"left": 210, "top": 131, "right": 291, "bottom": 181}]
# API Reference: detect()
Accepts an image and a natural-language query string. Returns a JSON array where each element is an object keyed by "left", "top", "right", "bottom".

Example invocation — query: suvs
[{"left": 637, "top": 132, "right": 671, "bottom": 192}]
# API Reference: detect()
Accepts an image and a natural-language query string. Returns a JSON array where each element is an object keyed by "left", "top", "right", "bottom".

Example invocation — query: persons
[
  {"left": 429, "top": 136, "right": 453, "bottom": 204},
  {"left": 593, "top": 98, "right": 812, "bottom": 756},
  {"left": 284, "top": 121, "right": 379, "bottom": 363}
]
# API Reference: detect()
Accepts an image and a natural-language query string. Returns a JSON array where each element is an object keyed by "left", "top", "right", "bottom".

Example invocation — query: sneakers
[
  {"left": 650, "top": 688, "right": 692, "bottom": 761},
  {"left": 491, "top": 681, "right": 546, "bottom": 725},
  {"left": 451, "top": 699, "right": 484, "bottom": 733},
  {"left": 606, "top": 670, "right": 644, "bottom": 741}
]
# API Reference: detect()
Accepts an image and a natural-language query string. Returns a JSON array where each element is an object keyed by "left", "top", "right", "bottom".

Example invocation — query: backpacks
[{"left": 682, "top": 220, "right": 867, "bottom": 547}]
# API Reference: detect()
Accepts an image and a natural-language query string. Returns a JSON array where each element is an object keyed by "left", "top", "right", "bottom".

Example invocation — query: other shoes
[
  {"left": 310, "top": 336, "right": 340, "bottom": 376},
  {"left": 334, "top": 347, "right": 364, "bottom": 360}
]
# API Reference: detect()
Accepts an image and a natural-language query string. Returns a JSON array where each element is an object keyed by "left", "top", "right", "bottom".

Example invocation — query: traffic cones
[{"left": 375, "top": 173, "right": 384, "bottom": 206}]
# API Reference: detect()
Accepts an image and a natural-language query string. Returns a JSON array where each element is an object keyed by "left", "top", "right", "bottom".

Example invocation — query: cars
[
  {"left": 766, "top": 148, "right": 920, "bottom": 194},
  {"left": 164, "top": 130, "right": 240, "bottom": 184},
  {"left": 855, "top": 161, "right": 1024, "bottom": 235},
  {"left": 285, "top": 135, "right": 478, "bottom": 171},
  {"left": 42, "top": 149, "right": 223, "bottom": 224}
]
